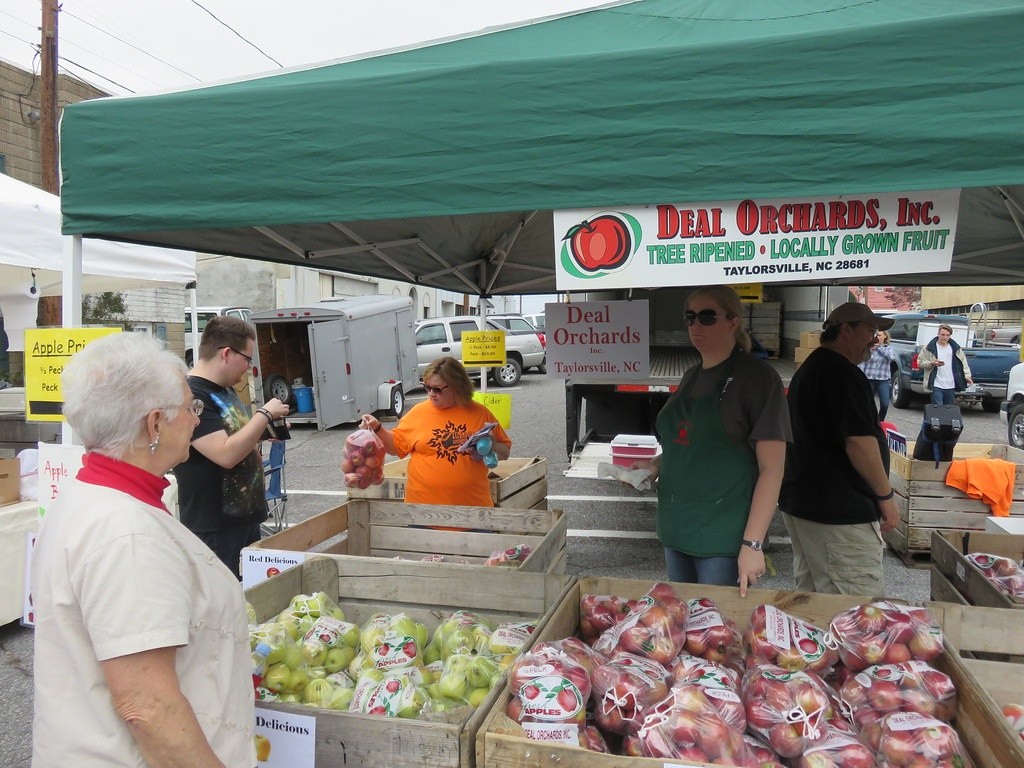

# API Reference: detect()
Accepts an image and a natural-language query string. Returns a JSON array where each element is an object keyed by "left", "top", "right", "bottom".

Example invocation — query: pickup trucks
[
  {"left": 877, "top": 313, "right": 1024, "bottom": 451},
  {"left": 414, "top": 311, "right": 546, "bottom": 390}
]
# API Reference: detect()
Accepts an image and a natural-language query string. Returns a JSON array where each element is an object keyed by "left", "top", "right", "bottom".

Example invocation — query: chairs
[{"left": 890, "top": 328, "right": 908, "bottom": 339}]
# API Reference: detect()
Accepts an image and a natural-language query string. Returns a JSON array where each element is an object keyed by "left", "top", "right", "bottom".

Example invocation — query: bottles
[
  {"left": 483, "top": 451, "right": 499, "bottom": 468},
  {"left": 475, "top": 437, "right": 492, "bottom": 455}
]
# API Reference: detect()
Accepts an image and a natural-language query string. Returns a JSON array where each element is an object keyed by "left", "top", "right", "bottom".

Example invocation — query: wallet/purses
[{"left": 259, "top": 416, "right": 293, "bottom": 443}]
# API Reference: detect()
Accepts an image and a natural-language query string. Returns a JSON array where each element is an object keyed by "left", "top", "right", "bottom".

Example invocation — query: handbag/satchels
[{"left": 339, "top": 423, "right": 387, "bottom": 492}]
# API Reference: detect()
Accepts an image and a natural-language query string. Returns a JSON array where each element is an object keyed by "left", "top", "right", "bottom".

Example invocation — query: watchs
[{"left": 742, "top": 539, "right": 763, "bottom": 551}]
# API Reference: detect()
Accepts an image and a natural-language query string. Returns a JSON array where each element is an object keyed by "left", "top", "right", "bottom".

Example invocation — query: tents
[
  {"left": 0, "top": 172, "right": 199, "bottom": 368},
  {"left": 59, "top": 0, "right": 1024, "bottom": 445}
]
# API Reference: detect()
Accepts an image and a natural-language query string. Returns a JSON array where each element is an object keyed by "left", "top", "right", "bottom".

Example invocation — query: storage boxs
[
  {"left": 244, "top": 562, "right": 577, "bottom": 768},
  {"left": 608, "top": 434, "right": 659, "bottom": 468},
  {"left": 930, "top": 533, "right": 1024, "bottom": 608},
  {"left": 239, "top": 499, "right": 568, "bottom": 589},
  {"left": 347, "top": 456, "right": 548, "bottom": 536},
  {"left": 986, "top": 516, "right": 1024, "bottom": 534},
  {"left": 477, "top": 578, "right": 1024, "bottom": 768},
  {"left": 880, "top": 442, "right": 1024, "bottom": 570},
  {"left": 0, "top": 457, "right": 21, "bottom": 508},
  {"left": 795, "top": 331, "right": 824, "bottom": 370},
  {"left": 729, "top": 283, "right": 782, "bottom": 360},
  {"left": 925, "top": 602, "right": 1024, "bottom": 768}
]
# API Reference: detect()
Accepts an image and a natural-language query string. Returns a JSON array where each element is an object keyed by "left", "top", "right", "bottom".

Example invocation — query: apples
[
  {"left": 246, "top": 592, "right": 522, "bottom": 719},
  {"left": 341, "top": 435, "right": 384, "bottom": 488},
  {"left": 267, "top": 568, "right": 280, "bottom": 578},
  {"left": 253, "top": 734, "right": 270, "bottom": 761},
  {"left": 981, "top": 558, "right": 1024, "bottom": 595},
  {"left": 1004, "top": 703, "right": 1024, "bottom": 719},
  {"left": 975, "top": 555, "right": 989, "bottom": 564},
  {"left": 505, "top": 583, "right": 974, "bottom": 768},
  {"left": 489, "top": 544, "right": 530, "bottom": 565}
]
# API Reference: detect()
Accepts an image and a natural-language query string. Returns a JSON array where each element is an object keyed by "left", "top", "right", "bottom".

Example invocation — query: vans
[{"left": 185, "top": 305, "right": 253, "bottom": 369}]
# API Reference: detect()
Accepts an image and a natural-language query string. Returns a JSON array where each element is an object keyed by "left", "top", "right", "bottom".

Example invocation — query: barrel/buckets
[{"left": 294, "top": 387, "right": 314, "bottom": 413}]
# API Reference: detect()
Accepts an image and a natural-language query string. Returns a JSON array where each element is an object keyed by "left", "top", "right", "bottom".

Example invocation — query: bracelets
[
  {"left": 255, "top": 408, "right": 273, "bottom": 423},
  {"left": 374, "top": 423, "right": 381, "bottom": 433},
  {"left": 878, "top": 488, "right": 895, "bottom": 500}
]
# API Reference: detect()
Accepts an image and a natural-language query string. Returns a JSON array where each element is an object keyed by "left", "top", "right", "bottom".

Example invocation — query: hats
[{"left": 822, "top": 299, "right": 895, "bottom": 334}]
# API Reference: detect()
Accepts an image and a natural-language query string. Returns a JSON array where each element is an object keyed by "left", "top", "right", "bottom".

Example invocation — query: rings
[{"left": 757, "top": 574, "right": 762, "bottom": 578}]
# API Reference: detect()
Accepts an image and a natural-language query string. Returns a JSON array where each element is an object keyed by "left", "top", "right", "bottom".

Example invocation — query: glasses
[
  {"left": 217, "top": 346, "right": 254, "bottom": 366},
  {"left": 679, "top": 308, "right": 722, "bottom": 325},
  {"left": 159, "top": 398, "right": 207, "bottom": 418},
  {"left": 421, "top": 380, "right": 450, "bottom": 394}
]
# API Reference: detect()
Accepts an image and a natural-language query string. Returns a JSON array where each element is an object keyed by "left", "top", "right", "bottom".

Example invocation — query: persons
[
  {"left": 778, "top": 302, "right": 900, "bottom": 597},
  {"left": 31, "top": 331, "right": 258, "bottom": 768},
  {"left": 616, "top": 285, "right": 793, "bottom": 598},
  {"left": 863, "top": 330, "right": 896, "bottom": 422},
  {"left": 358, "top": 357, "right": 513, "bottom": 532},
  {"left": 916, "top": 323, "right": 973, "bottom": 405},
  {"left": 173, "top": 315, "right": 292, "bottom": 582}
]
